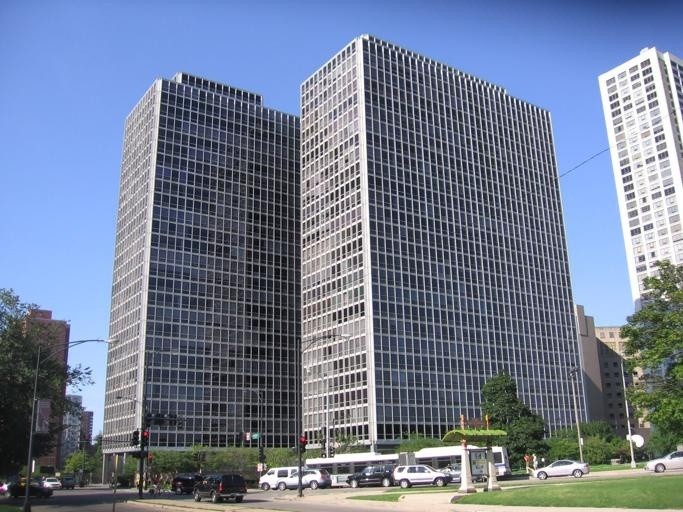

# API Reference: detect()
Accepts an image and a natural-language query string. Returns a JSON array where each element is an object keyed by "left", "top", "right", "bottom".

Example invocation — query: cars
[
  {"left": 345, "top": 462, "right": 481, "bottom": 488},
  {"left": 642, "top": 450, "right": 682, "bottom": 473},
  {"left": 0, "top": 476, "right": 76, "bottom": 499},
  {"left": 532, "top": 459, "right": 591, "bottom": 480}
]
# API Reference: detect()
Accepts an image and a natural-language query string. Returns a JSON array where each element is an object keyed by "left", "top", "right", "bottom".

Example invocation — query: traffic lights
[
  {"left": 141, "top": 431, "right": 149, "bottom": 446},
  {"left": 177, "top": 415, "right": 183, "bottom": 431},
  {"left": 258, "top": 446, "right": 263, "bottom": 461},
  {"left": 132, "top": 430, "right": 140, "bottom": 445},
  {"left": 299, "top": 434, "right": 307, "bottom": 453}
]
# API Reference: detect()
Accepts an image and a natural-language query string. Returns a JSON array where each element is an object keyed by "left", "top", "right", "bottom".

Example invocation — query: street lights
[
  {"left": 241, "top": 388, "right": 264, "bottom": 478},
  {"left": 297, "top": 332, "right": 352, "bottom": 498},
  {"left": 114, "top": 396, "right": 149, "bottom": 491},
  {"left": 568, "top": 365, "right": 584, "bottom": 463},
  {"left": 20, "top": 337, "right": 120, "bottom": 512}
]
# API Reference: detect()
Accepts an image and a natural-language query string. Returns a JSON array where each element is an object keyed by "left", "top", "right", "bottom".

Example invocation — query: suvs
[{"left": 170, "top": 468, "right": 333, "bottom": 505}]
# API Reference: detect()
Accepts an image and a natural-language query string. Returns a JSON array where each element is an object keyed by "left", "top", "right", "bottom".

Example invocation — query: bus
[{"left": 305, "top": 443, "right": 515, "bottom": 489}]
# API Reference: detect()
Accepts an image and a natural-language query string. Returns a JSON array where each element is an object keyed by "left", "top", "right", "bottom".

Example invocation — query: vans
[{"left": 257, "top": 466, "right": 308, "bottom": 490}]
